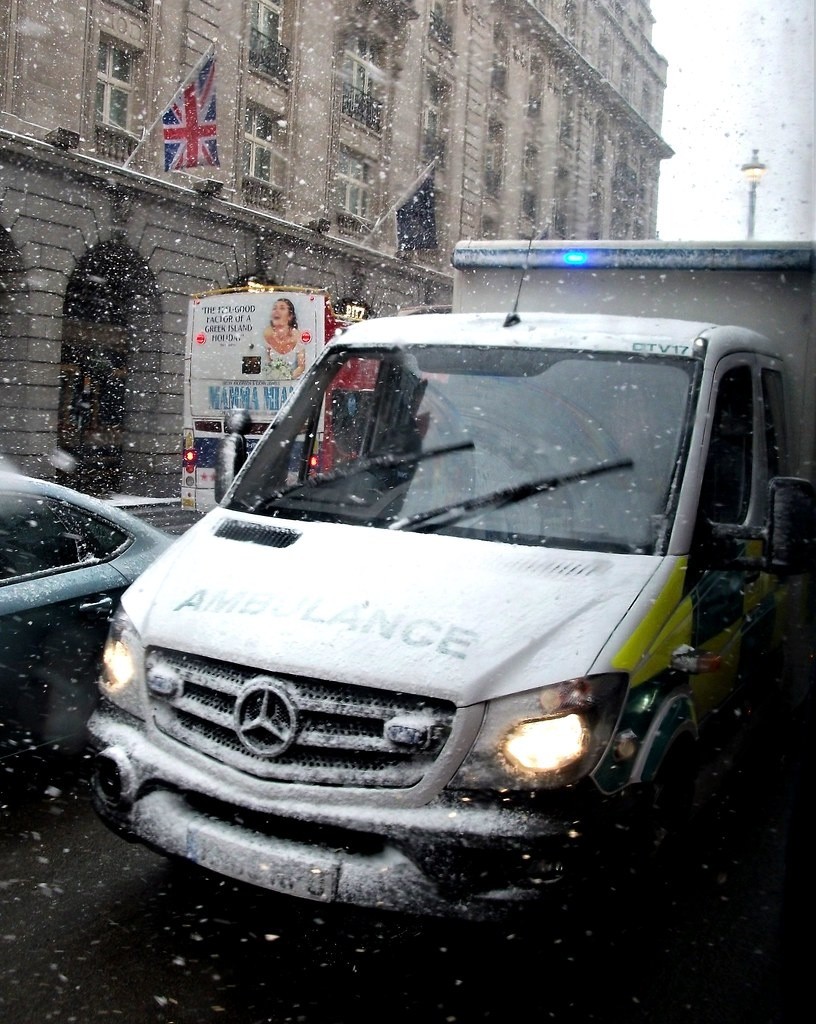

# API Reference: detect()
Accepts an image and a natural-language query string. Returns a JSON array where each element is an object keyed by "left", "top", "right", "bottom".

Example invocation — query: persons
[{"left": 260, "top": 299, "right": 305, "bottom": 379}]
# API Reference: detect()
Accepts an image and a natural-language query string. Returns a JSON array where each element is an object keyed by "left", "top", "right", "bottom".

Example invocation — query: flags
[
  {"left": 162, "top": 52, "right": 220, "bottom": 174},
  {"left": 395, "top": 167, "right": 439, "bottom": 250}
]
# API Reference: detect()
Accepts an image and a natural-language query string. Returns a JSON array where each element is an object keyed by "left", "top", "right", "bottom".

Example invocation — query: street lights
[{"left": 741, "top": 147, "right": 769, "bottom": 238}]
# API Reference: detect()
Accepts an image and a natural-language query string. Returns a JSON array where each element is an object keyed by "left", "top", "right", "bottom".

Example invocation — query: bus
[{"left": 181, "top": 285, "right": 366, "bottom": 513}]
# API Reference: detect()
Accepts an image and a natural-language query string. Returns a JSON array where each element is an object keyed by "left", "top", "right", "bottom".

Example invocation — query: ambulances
[{"left": 83, "top": 239, "right": 815, "bottom": 969}]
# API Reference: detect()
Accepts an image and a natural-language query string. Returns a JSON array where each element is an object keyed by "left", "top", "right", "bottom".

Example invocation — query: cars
[
  {"left": 0, "top": 472, "right": 181, "bottom": 801},
  {"left": 388, "top": 305, "right": 452, "bottom": 317}
]
